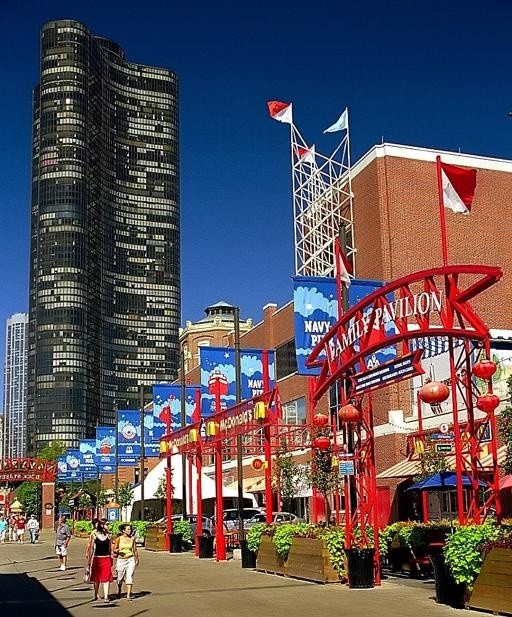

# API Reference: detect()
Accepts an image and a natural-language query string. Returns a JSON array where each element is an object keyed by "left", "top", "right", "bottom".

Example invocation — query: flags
[
  {"left": 290, "top": 271, "right": 343, "bottom": 379},
  {"left": 237, "top": 347, "right": 279, "bottom": 402},
  {"left": 295, "top": 142, "right": 319, "bottom": 165},
  {"left": 436, "top": 154, "right": 479, "bottom": 217},
  {"left": 265, "top": 98, "right": 295, "bottom": 124},
  {"left": 333, "top": 238, "right": 355, "bottom": 292},
  {"left": 195, "top": 342, "right": 238, "bottom": 441},
  {"left": 345, "top": 276, "right": 398, "bottom": 370},
  {"left": 323, "top": 105, "right": 350, "bottom": 134}
]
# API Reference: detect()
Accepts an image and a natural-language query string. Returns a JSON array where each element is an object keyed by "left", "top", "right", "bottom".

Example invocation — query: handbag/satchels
[{"left": 84, "top": 565, "right": 91, "bottom": 582}]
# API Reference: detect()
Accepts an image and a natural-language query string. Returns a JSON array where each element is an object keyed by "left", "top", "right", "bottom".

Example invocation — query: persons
[
  {"left": 113, "top": 523, "right": 140, "bottom": 602},
  {"left": 0, "top": 507, "right": 42, "bottom": 545},
  {"left": 56, "top": 382, "right": 204, "bottom": 485},
  {"left": 53, "top": 515, "right": 72, "bottom": 572},
  {"left": 91, "top": 519, "right": 99, "bottom": 535},
  {"left": 86, "top": 517, "right": 114, "bottom": 602}
]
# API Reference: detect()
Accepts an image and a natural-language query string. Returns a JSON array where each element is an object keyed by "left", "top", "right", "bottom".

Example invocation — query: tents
[{"left": 118, "top": 444, "right": 259, "bottom": 521}]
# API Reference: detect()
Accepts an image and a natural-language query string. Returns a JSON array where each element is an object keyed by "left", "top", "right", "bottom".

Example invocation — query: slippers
[{"left": 95, "top": 593, "right": 133, "bottom": 603}]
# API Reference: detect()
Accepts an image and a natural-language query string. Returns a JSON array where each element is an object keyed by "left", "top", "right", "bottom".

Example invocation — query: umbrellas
[
  {"left": 482, "top": 473, "right": 511, "bottom": 495},
  {"left": 405, "top": 471, "right": 488, "bottom": 521}
]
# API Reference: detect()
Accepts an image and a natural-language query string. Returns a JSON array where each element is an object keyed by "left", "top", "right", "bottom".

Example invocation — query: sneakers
[{"left": 59, "top": 563, "right": 66, "bottom": 571}]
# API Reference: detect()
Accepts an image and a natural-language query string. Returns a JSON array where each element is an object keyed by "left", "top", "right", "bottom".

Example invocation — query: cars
[{"left": 128, "top": 505, "right": 304, "bottom": 545}]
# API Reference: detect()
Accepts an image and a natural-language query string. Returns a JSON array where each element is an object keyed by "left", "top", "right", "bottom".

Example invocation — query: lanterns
[
  {"left": 313, "top": 434, "right": 332, "bottom": 455},
  {"left": 339, "top": 403, "right": 360, "bottom": 425},
  {"left": 312, "top": 411, "right": 329, "bottom": 429},
  {"left": 472, "top": 358, "right": 497, "bottom": 383},
  {"left": 475, "top": 392, "right": 500, "bottom": 419},
  {"left": 417, "top": 377, "right": 450, "bottom": 416}
]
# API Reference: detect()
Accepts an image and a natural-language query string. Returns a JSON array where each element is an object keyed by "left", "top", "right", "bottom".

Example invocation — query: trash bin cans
[
  {"left": 197, "top": 535, "right": 215, "bottom": 558},
  {"left": 168, "top": 533, "right": 184, "bottom": 553},
  {"left": 343, "top": 548, "right": 376, "bottom": 588},
  {"left": 428, "top": 554, "right": 465, "bottom": 609},
  {"left": 239, "top": 540, "right": 260, "bottom": 568}
]
